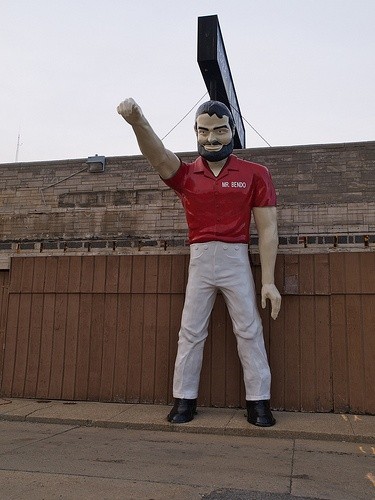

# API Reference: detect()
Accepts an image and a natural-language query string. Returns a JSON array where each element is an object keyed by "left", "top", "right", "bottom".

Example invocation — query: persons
[{"left": 114, "top": 96, "right": 282, "bottom": 428}]
